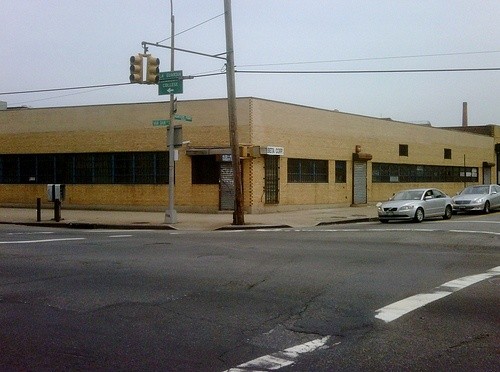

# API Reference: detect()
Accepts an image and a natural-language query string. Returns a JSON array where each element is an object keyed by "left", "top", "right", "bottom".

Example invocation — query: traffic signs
[{"left": 158, "top": 70, "right": 184, "bottom": 96}]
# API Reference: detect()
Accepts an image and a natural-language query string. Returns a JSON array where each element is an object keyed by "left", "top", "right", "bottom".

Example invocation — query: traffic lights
[
  {"left": 129, "top": 53, "right": 144, "bottom": 84},
  {"left": 146, "top": 55, "right": 161, "bottom": 85}
]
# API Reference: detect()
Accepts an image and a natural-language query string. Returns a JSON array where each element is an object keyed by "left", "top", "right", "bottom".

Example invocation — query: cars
[
  {"left": 452, "top": 184, "right": 500, "bottom": 214},
  {"left": 377, "top": 188, "right": 456, "bottom": 223}
]
